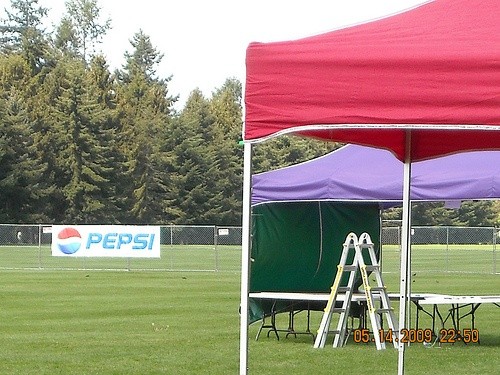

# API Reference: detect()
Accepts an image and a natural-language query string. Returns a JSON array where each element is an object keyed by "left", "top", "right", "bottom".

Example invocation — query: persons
[{"left": 17, "top": 229, "right": 22, "bottom": 245}]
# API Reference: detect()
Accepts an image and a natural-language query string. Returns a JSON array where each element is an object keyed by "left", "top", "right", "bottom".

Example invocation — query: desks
[{"left": 249, "top": 290, "right": 500, "bottom": 346}]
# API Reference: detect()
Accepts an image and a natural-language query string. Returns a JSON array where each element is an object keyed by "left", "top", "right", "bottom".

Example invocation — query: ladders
[{"left": 313, "top": 232, "right": 401, "bottom": 351}]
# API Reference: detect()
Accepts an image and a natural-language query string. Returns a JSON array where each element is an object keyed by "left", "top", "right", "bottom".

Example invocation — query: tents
[
  {"left": 238, "top": 142, "right": 500, "bottom": 325},
  {"left": 239, "top": 1, "right": 500, "bottom": 375}
]
